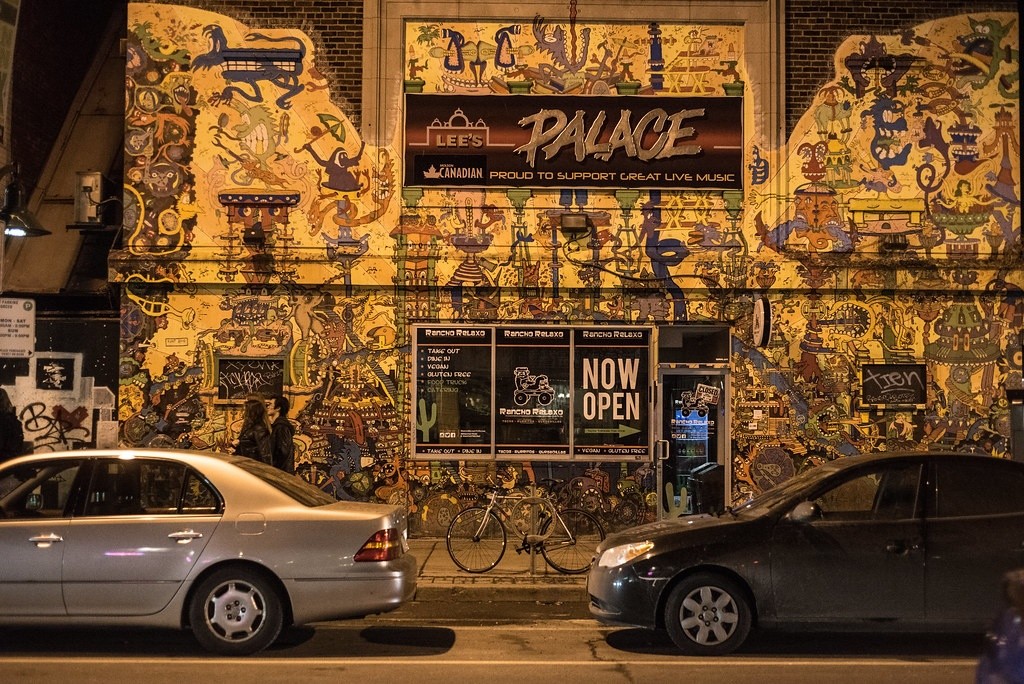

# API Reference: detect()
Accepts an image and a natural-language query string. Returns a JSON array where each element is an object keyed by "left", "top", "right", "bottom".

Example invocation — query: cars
[
  {"left": 0, "top": 446, "right": 413, "bottom": 655},
  {"left": 579, "top": 447, "right": 1024, "bottom": 660}
]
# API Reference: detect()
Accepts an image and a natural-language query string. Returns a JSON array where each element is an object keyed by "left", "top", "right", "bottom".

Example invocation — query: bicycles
[{"left": 444, "top": 478, "right": 605, "bottom": 576}]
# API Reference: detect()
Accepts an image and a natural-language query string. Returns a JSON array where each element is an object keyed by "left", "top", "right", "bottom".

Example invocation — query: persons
[
  {"left": 266, "top": 396, "right": 296, "bottom": 476},
  {"left": 231, "top": 399, "right": 273, "bottom": 467}
]
